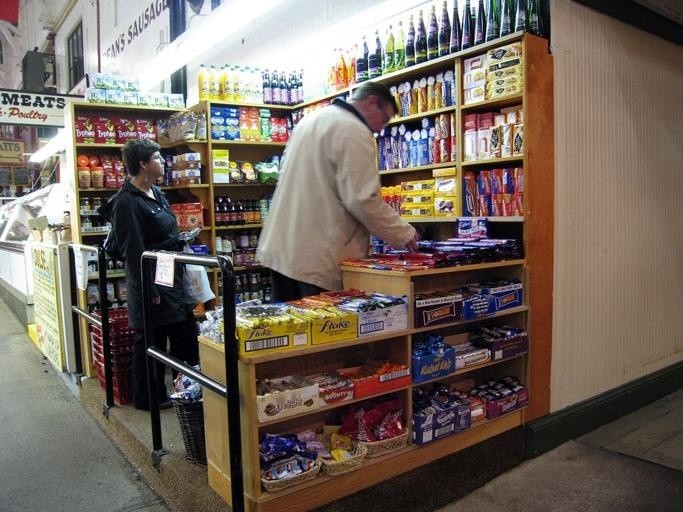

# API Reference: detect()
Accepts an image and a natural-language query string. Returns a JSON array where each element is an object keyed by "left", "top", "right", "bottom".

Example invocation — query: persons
[
  {"left": 253, "top": 82, "right": 419, "bottom": 302},
  {"left": 100, "top": 138, "right": 199, "bottom": 411}
]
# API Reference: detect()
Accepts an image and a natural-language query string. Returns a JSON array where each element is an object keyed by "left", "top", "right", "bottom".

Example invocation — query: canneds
[
  {"left": 215, "top": 229, "right": 261, "bottom": 267},
  {"left": 80, "top": 196, "right": 111, "bottom": 232}
]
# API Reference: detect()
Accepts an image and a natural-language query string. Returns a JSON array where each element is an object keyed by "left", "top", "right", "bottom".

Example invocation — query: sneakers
[{"left": 159, "top": 401, "right": 174, "bottom": 409}]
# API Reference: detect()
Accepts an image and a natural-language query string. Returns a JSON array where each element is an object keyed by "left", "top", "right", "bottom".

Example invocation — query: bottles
[
  {"left": 215, "top": 196, "right": 266, "bottom": 226},
  {"left": 197, "top": 64, "right": 305, "bottom": 105},
  {"left": 328, "top": 0, "right": 543, "bottom": 89},
  {"left": 218, "top": 275, "right": 272, "bottom": 306}
]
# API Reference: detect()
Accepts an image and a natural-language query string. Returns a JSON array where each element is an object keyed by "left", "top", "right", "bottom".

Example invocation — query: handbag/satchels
[
  {"left": 102, "top": 227, "right": 125, "bottom": 261},
  {"left": 181, "top": 242, "right": 216, "bottom": 305}
]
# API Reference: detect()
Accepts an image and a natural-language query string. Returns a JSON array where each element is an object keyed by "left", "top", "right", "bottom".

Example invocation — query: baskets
[
  {"left": 168, "top": 396, "right": 207, "bottom": 471},
  {"left": 261, "top": 428, "right": 409, "bottom": 493},
  {"left": 87, "top": 307, "right": 135, "bottom": 407}
]
